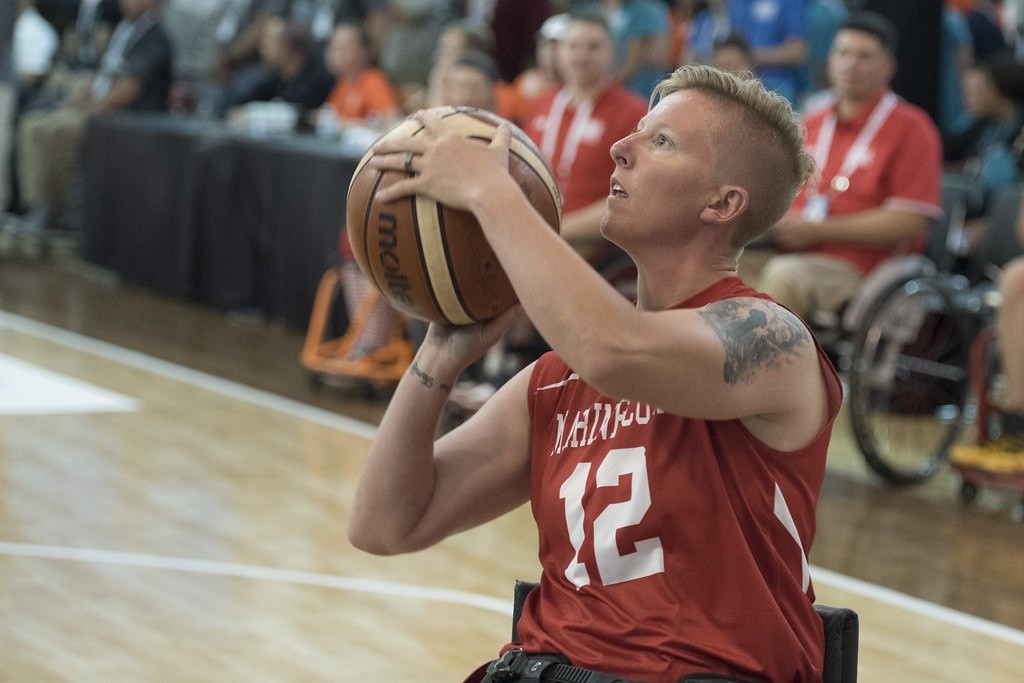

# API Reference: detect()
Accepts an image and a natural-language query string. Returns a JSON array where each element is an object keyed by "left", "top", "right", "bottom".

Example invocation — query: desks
[{"left": 82, "top": 115, "right": 373, "bottom": 338}]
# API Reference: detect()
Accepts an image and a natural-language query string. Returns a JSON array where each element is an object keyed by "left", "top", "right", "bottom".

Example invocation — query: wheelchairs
[{"left": 786, "top": 181, "right": 980, "bottom": 488}]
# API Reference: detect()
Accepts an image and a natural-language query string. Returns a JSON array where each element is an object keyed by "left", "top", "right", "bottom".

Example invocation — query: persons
[
  {"left": 0, "top": 0, "right": 1023, "bottom": 454},
  {"left": 349, "top": 63, "right": 845, "bottom": 682}
]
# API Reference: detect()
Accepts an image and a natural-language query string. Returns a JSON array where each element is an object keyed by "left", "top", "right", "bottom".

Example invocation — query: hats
[{"left": 539, "top": 12, "right": 572, "bottom": 40}]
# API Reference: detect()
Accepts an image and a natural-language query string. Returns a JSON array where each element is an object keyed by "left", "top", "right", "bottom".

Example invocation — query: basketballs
[{"left": 343, "top": 105, "right": 566, "bottom": 325}]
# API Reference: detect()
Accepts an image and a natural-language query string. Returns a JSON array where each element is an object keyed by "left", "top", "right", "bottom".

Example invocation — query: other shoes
[{"left": 948, "top": 434, "right": 1024, "bottom": 474}]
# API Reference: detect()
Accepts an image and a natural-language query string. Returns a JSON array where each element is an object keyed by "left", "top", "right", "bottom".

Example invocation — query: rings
[{"left": 405, "top": 152, "right": 416, "bottom": 172}]
